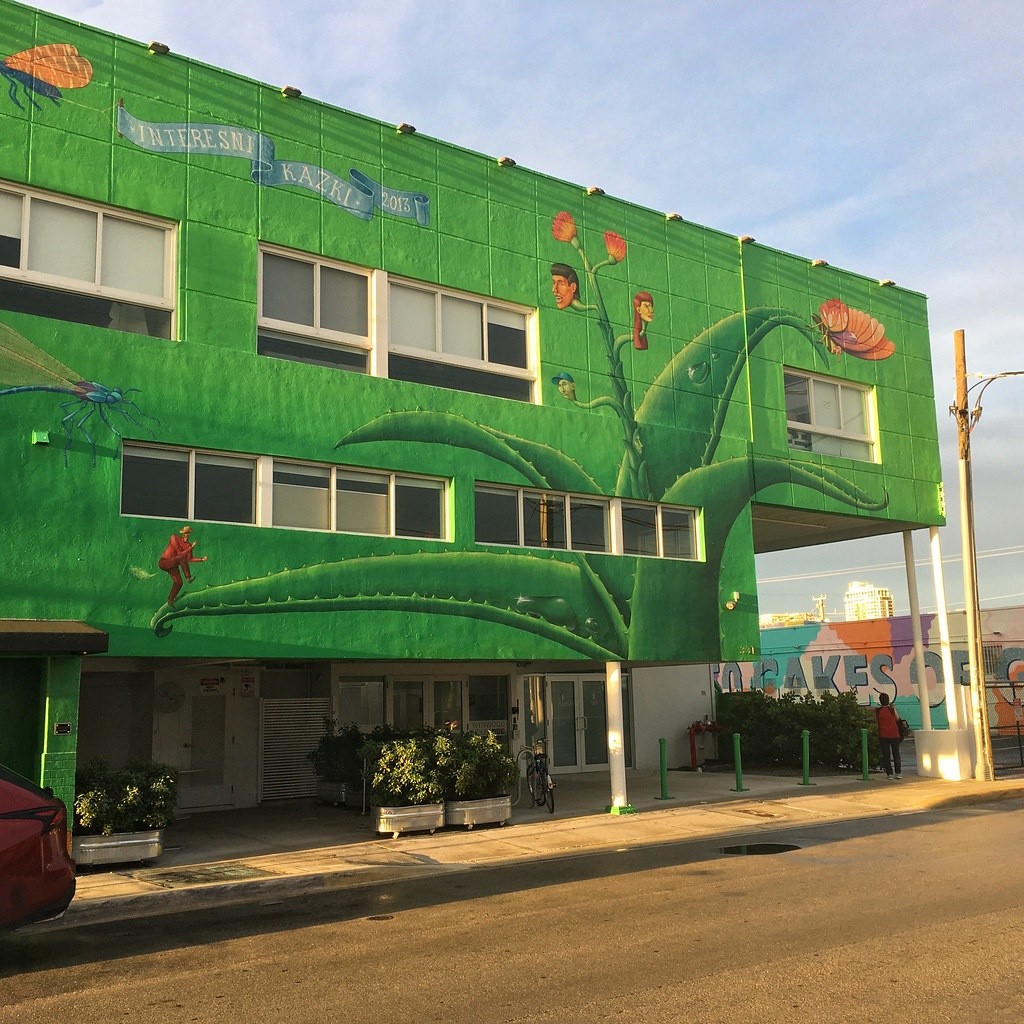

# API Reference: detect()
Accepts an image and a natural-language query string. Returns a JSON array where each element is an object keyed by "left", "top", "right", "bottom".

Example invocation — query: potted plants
[
  {"left": 72, "top": 753, "right": 177, "bottom": 866},
  {"left": 306, "top": 710, "right": 522, "bottom": 835}
]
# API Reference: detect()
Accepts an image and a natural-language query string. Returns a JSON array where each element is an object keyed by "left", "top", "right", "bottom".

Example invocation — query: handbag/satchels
[{"left": 897, "top": 719, "right": 909, "bottom": 742}]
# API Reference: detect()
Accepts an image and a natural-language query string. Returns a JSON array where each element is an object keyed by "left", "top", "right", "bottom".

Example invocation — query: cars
[{"left": 1, "top": 761, "right": 79, "bottom": 938}]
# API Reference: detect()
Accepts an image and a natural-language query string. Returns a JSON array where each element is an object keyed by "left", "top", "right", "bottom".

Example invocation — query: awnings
[{"left": 0, "top": 620, "right": 109, "bottom": 656}]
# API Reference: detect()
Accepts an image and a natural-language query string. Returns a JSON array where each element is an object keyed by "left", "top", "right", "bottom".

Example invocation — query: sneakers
[
  {"left": 888, "top": 774, "right": 894, "bottom": 780},
  {"left": 896, "top": 773, "right": 902, "bottom": 779}
]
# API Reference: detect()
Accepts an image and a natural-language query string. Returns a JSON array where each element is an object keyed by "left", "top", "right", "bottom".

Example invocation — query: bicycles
[{"left": 518, "top": 738, "right": 557, "bottom": 815}]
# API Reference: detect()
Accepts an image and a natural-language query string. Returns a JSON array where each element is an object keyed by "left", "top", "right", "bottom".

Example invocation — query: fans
[{"left": 153, "top": 682, "right": 186, "bottom": 713}]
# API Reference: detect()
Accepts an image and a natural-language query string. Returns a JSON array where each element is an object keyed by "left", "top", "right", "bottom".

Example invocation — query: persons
[{"left": 874, "top": 693, "right": 902, "bottom": 779}]
